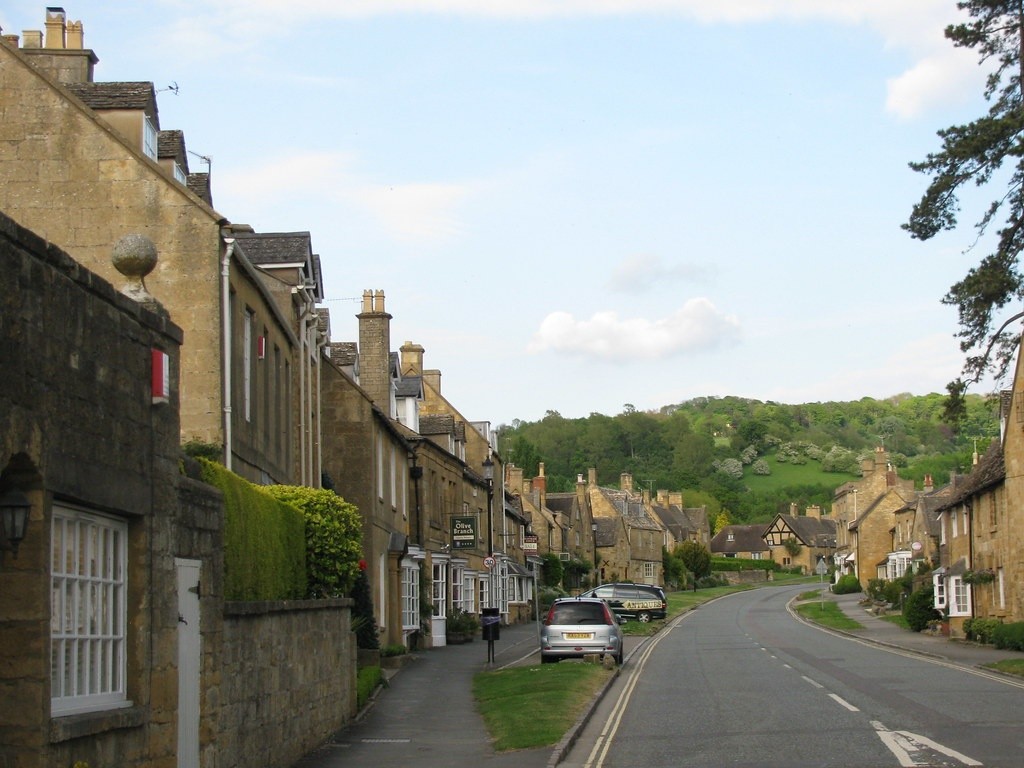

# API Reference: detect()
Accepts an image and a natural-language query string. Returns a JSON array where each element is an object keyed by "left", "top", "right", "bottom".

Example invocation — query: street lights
[
  {"left": 482, "top": 454, "right": 495, "bottom": 557},
  {"left": 693, "top": 537, "right": 696, "bottom": 592},
  {"left": 591, "top": 520, "right": 598, "bottom": 588}
]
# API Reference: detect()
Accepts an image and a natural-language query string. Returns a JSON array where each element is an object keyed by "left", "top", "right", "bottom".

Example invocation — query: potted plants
[
  {"left": 446, "top": 607, "right": 479, "bottom": 644},
  {"left": 416, "top": 561, "right": 436, "bottom": 649}
]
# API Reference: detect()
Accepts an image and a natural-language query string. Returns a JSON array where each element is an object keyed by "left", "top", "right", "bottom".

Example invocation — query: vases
[
  {"left": 930, "top": 625, "right": 936, "bottom": 631},
  {"left": 936, "top": 625, "right": 942, "bottom": 631},
  {"left": 358, "top": 649, "right": 381, "bottom": 669}
]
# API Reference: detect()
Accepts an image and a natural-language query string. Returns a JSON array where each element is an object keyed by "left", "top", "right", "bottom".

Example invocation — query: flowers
[
  {"left": 926, "top": 619, "right": 943, "bottom": 627},
  {"left": 350, "top": 561, "right": 381, "bottom": 649},
  {"left": 960, "top": 568, "right": 996, "bottom": 584}
]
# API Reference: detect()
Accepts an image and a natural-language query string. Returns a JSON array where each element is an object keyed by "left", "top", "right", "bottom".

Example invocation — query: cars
[{"left": 540, "top": 597, "right": 627, "bottom": 665}]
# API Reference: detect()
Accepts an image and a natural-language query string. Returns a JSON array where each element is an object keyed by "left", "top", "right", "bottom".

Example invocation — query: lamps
[{"left": 0, "top": 487, "right": 33, "bottom": 561}]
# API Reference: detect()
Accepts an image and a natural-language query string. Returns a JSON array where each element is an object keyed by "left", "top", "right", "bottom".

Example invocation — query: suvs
[{"left": 577, "top": 582, "right": 668, "bottom": 623}]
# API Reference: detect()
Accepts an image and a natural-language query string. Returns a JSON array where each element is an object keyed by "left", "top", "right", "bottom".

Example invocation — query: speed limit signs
[{"left": 483, "top": 557, "right": 496, "bottom": 569}]
